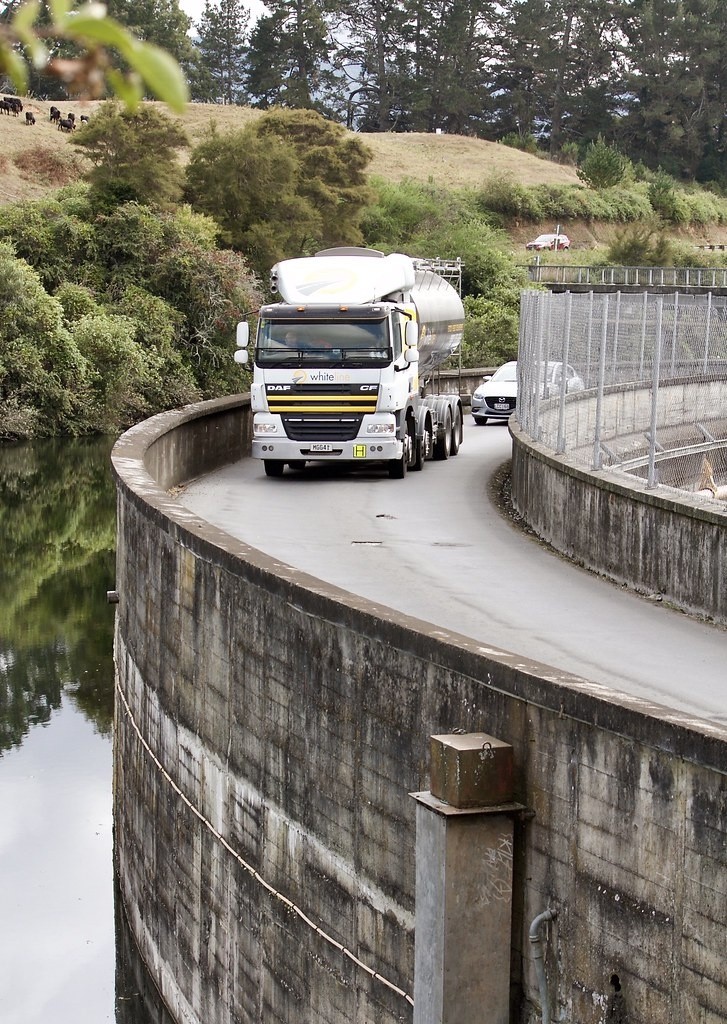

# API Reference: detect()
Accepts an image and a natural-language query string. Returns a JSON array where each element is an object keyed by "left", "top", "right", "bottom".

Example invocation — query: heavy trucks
[{"left": 235, "top": 246, "right": 467, "bottom": 481}]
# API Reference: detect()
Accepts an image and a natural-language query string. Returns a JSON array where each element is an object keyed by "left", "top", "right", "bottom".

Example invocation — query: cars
[
  {"left": 526, "top": 233, "right": 570, "bottom": 253},
  {"left": 471, "top": 360, "right": 584, "bottom": 424}
]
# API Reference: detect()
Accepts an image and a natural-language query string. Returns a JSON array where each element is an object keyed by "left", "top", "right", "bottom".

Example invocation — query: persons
[
  {"left": 285, "top": 331, "right": 299, "bottom": 347},
  {"left": 53, "top": 108, "right": 58, "bottom": 123}
]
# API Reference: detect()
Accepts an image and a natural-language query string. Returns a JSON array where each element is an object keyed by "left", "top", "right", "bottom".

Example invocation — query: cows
[{"left": 0, "top": 97, "right": 89, "bottom": 133}]
